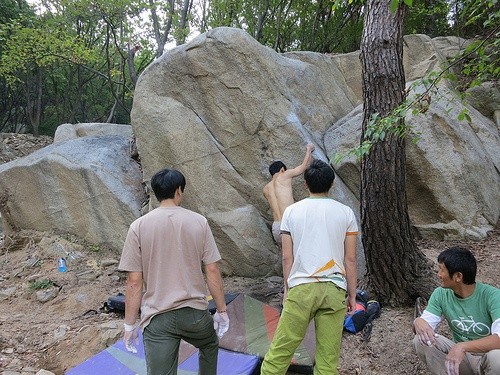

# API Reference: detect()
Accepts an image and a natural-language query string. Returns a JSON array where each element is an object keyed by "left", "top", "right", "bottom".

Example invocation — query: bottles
[{"left": 57, "top": 257, "right": 67, "bottom": 272}]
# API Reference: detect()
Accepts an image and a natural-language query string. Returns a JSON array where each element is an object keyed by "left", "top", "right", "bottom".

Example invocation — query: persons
[
  {"left": 412, "top": 247, "right": 500, "bottom": 375},
  {"left": 117, "top": 169, "right": 231, "bottom": 375},
  {"left": 263, "top": 141, "right": 316, "bottom": 250},
  {"left": 260, "top": 158, "right": 358, "bottom": 375}
]
